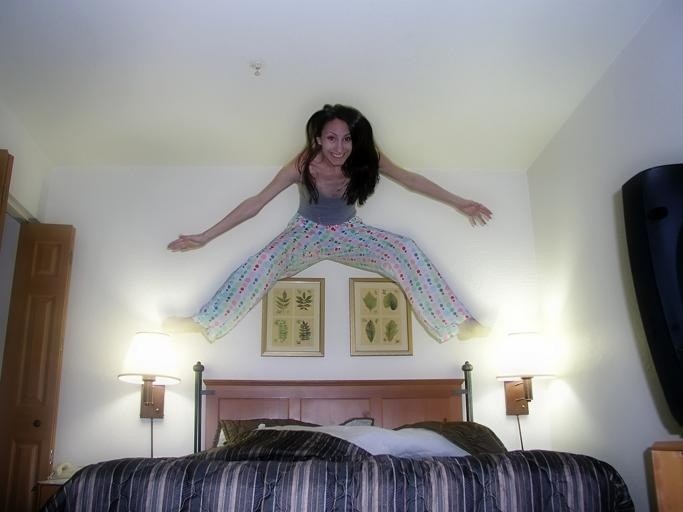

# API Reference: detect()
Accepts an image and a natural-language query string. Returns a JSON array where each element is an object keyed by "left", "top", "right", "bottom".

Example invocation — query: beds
[{"left": 39, "top": 360, "right": 636, "bottom": 511}]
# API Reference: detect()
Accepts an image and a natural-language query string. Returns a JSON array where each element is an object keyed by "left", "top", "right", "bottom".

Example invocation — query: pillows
[
  {"left": 217, "top": 415, "right": 322, "bottom": 449},
  {"left": 392, "top": 421, "right": 508, "bottom": 456}
]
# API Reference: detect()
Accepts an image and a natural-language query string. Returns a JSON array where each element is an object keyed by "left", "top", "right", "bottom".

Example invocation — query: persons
[{"left": 167, "top": 103, "right": 492, "bottom": 346}]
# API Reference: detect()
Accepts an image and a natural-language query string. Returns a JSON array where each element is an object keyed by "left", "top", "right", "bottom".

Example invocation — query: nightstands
[
  {"left": 36, "top": 480, "right": 68, "bottom": 512},
  {"left": 651, "top": 440, "right": 682, "bottom": 510}
]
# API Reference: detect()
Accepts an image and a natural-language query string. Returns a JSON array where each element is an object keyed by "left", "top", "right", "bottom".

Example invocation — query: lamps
[
  {"left": 117, "top": 331, "right": 182, "bottom": 418},
  {"left": 496, "top": 329, "right": 554, "bottom": 416}
]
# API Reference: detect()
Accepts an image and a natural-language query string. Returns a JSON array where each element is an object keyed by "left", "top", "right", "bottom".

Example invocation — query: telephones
[{"left": 53, "top": 463, "right": 75, "bottom": 479}]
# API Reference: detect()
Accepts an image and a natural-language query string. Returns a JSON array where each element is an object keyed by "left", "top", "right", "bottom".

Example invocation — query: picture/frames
[
  {"left": 348, "top": 276, "right": 413, "bottom": 357},
  {"left": 260, "top": 277, "right": 325, "bottom": 357}
]
